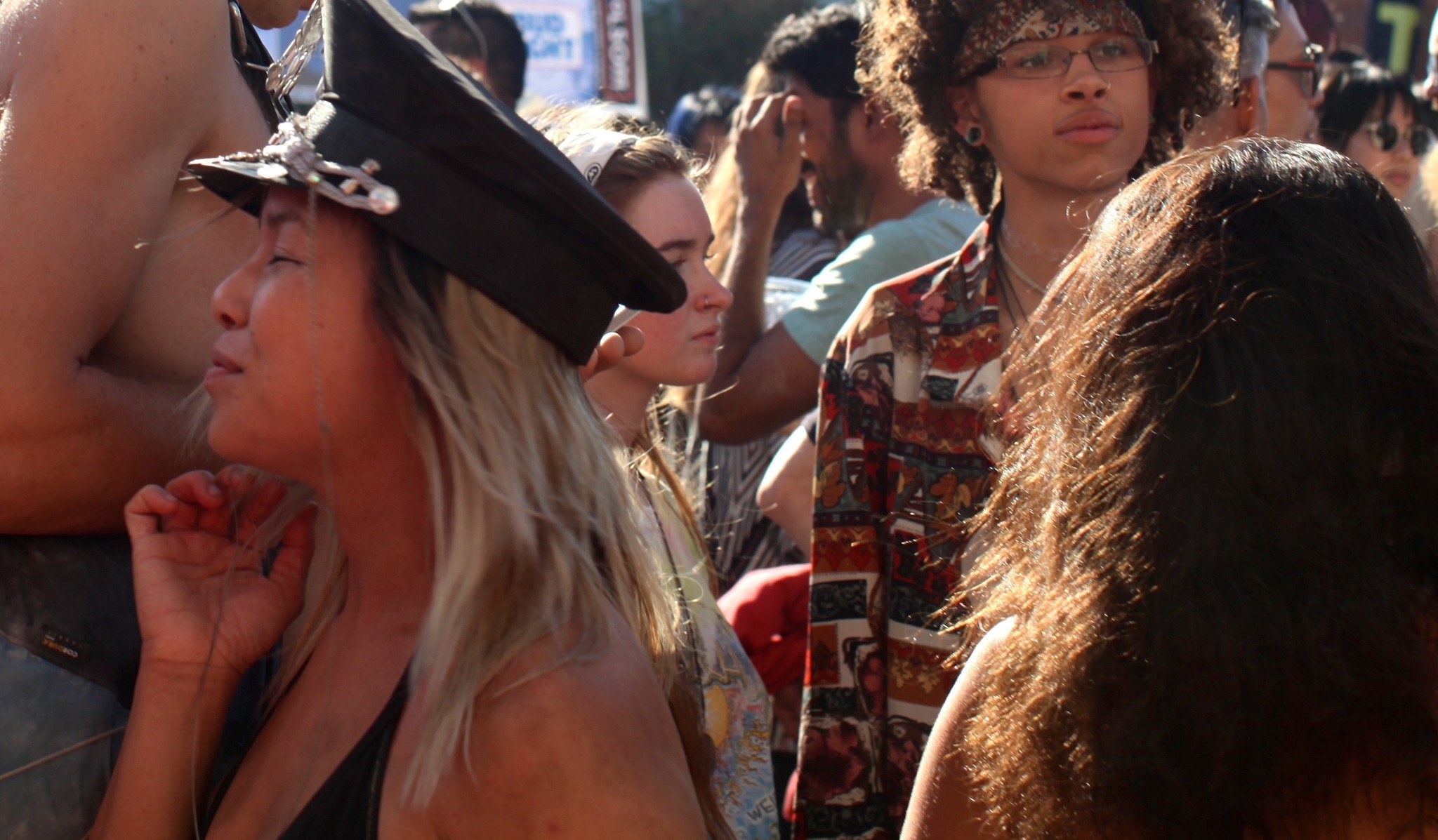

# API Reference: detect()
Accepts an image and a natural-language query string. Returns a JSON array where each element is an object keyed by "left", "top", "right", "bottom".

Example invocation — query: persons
[
  {"left": 407, "top": 0, "right": 1438, "bottom": 840},
  {"left": 0, "top": 0, "right": 318, "bottom": 840},
  {"left": 86, "top": 0, "right": 704, "bottom": 840}
]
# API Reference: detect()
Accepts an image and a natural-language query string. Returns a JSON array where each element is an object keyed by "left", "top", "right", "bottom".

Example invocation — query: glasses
[
  {"left": 1358, "top": 117, "right": 1435, "bottom": 158},
  {"left": 1266, "top": 45, "right": 1328, "bottom": 97},
  {"left": 958, "top": 32, "right": 1162, "bottom": 80}
]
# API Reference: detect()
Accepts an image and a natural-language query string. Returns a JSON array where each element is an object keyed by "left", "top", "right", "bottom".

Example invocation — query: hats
[{"left": 188, "top": 0, "right": 690, "bottom": 364}]
